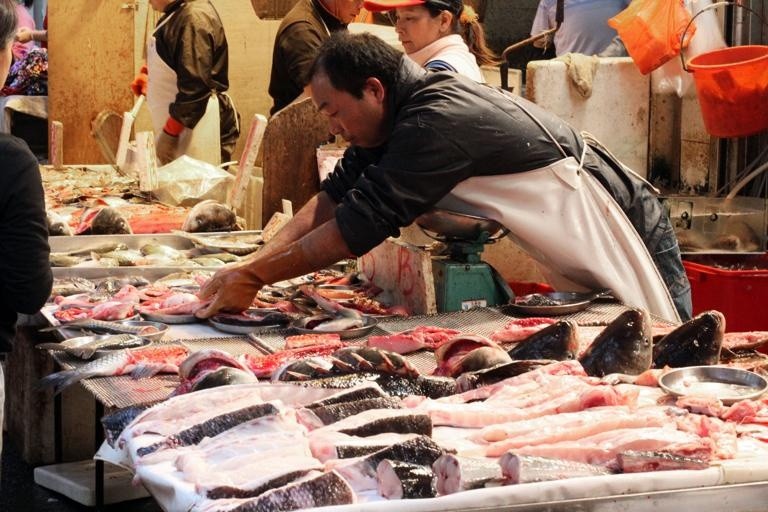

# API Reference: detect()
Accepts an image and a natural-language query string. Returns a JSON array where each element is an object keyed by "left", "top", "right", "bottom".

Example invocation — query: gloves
[
  {"left": 131, "top": 73, "right": 148, "bottom": 97},
  {"left": 195, "top": 242, "right": 312, "bottom": 319},
  {"left": 155, "top": 130, "right": 179, "bottom": 165}
]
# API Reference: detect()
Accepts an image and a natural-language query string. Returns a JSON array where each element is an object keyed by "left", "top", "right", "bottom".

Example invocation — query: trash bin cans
[{"left": 683, "top": 255, "right": 768, "bottom": 336}]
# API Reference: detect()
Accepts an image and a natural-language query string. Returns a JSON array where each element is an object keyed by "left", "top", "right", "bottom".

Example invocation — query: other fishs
[
  {"left": 507, "top": 319, "right": 581, "bottom": 362},
  {"left": 75, "top": 206, "right": 133, "bottom": 235},
  {"left": 44, "top": 207, "right": 69, "bottom": 236},
  {"left": 653, "top": 311, "right": 725, "bottom": 369},
  {"left": 42, "top": 348, "right": 140, "bottom": 403},
  {"left": 514, "top": 287, "right": 617, "bottom": 307},
  {"left": 180, "top": 198, "right": 245, "bottom": 233},
  {"left": 579, "top": 307, "right": 653, "bottom": 377},
  {"left": 432, "top": 332, "right": 515, "bottom": 378},
  {"left": 47, "top": 235, "right": 266, "bottom": 270},
  {"left": 41, "top": 319, "right": 159, "bottom": 336}
]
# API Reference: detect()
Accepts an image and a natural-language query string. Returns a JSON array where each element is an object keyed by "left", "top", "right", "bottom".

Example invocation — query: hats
[{"left": 362, "top": 0, "right": 464, "bottom": 14}]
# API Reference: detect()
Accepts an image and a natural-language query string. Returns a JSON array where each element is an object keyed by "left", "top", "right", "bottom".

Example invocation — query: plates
[
  {"left": 415, "top": 207, "right": 506, "bottom": 241},
  {"left": 209, "top": 307, "right": 291, "bottom": 336},
  {"left": 59, "top": 335, "right": 153, "bottom": 356},
  {"left": 82, "top": 320, "right": 169, "bottom": 341},
  {"left": 294, "top": 312, "right": 377, "bottom": 339},
  {"left": 298, "top": 285, "right": 369, "bottom": 306},
  {"left": 509, "top": 292, "right": 591, "bottom": 315},
  {"left": 138, "top": 310, "right": 209, "bottom": 325},
  {"left": 658, "top": 366, "right": 767, "bottom": 406},
  {"left": 43, "top": 231, "right": 265, "bottom": 281}
]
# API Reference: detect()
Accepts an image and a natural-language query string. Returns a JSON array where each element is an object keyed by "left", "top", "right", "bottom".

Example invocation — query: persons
[
  {"left": 2, "top": 1, "right": 52, "bottom": 510},
  {"left": 268, "top": 0, "right": 363, "bottom": 116},
  {"left": 148, "top": 0, "right": 244, "bottom": 170},
  {"left": 15, "top": 11, "right": 49, "bottom": 46},
  {"left": 530, "top": 0, "right": 627, "bottom": 56},
  {"left": 473, "top": 0, "right": 538, "bottom": 58},
  {"left": 363, "top": 1, "right": 492, "bottom": 84},
  {"left": 197, "top": 33, "right": 693, "bottom": 326},
  {"left": 10, "top": 1, "right": 34, "bottom": 64}
]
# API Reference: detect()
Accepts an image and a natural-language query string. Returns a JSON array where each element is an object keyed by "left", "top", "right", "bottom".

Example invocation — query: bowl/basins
[{"left": 149, "top": 153, "right": 231, "bottom": 207}]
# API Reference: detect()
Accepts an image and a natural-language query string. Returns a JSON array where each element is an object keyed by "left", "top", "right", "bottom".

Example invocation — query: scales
[{"left": 417, "top": 208, "right": 515, "bottom": 311}]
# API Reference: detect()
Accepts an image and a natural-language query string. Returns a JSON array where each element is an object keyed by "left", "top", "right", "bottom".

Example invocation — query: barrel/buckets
[{"left": 679, "top": 0, "right": 768, "bottom": 138}]
